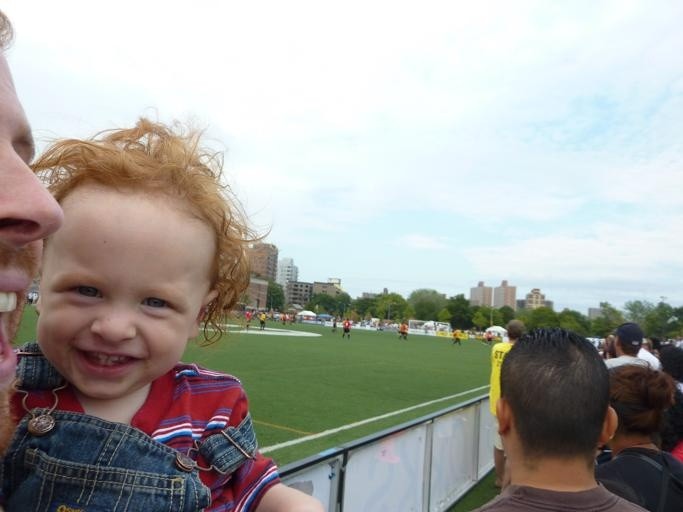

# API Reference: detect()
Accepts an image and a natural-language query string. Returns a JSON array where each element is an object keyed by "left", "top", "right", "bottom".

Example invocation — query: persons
[
  {"left": 0, "top": 9, "right": 64, "bottom": 458},
  {"left": 245, "top": 310, "right": 682, "bottom": 511},
  {"left": 0, "top": 119, "right": 329, "bottom": 512}
]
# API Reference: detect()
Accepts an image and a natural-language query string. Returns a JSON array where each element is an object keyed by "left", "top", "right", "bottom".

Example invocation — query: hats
[{"left": 614, "top": 322, "right": 642, "bottom": 346}]
